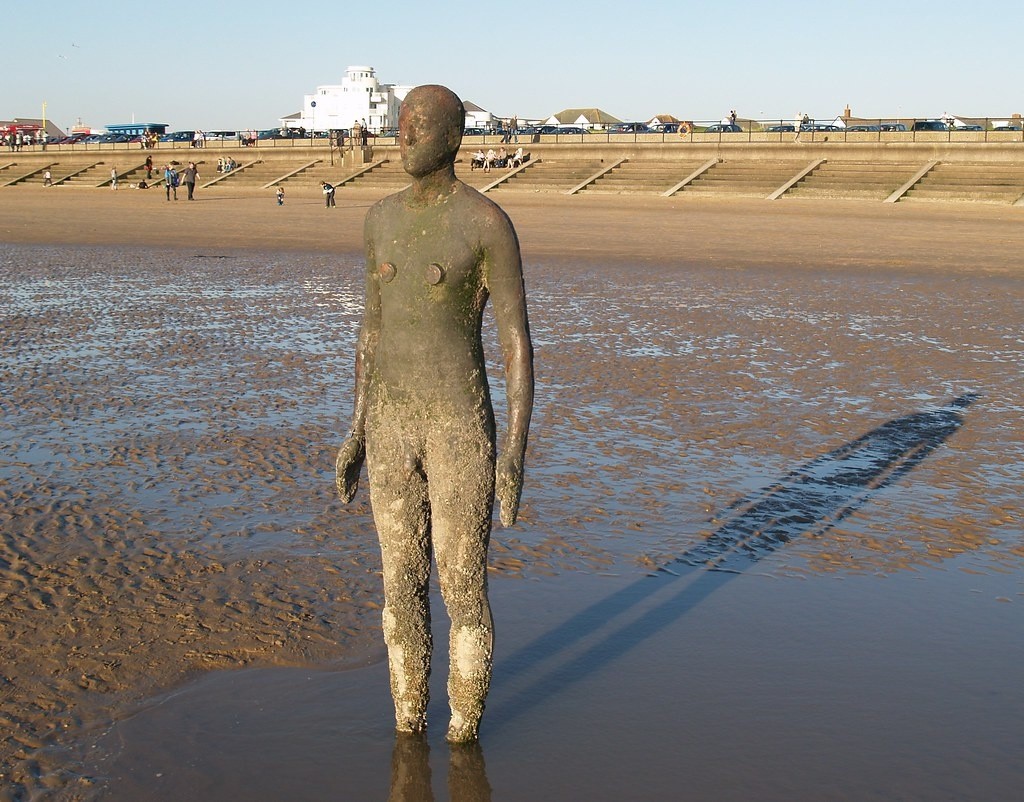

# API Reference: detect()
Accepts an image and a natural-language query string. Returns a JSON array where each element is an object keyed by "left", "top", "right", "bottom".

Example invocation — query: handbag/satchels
[
  {"left": 173, "top": 178, "right": 180, "bottom": 187},
  {"left": 494, "top": 159, "right": 501, "bottom": 168},
  {"left": 237, "top": 163, "right": 241, "bottom": 167}
]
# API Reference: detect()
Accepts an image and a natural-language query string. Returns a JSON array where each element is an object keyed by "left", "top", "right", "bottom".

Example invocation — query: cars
[
  {"left": 704, "top": 124, "right": 743, "bottom": 132},
  {"left": 47, "top": 121, "right": 590, "bottom": 140},
  {"left": 606, "top": 122, "right": 655, "bottom": 134},
  {"left": 647, "top": 123, "right": 687, "bottom": 133},
  {"left": 911, "top": 122, "right": 950, "bottom": 132},
  {"left": 957, "top": 125, "right": 982, "bottom": 131},
  {"left": 993, "top": 126, "right": 1021, "bottom": 131},
  {"left": 765, "top": 126, "right": 795, "bottom": 132},
  {"left": 799, "top": 123, "right": 906, "bottom": 132}
]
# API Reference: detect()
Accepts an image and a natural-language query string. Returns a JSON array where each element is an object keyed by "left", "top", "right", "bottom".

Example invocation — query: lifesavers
[{"left": 677, "top": 123, "right": 691, "bottom": 137}]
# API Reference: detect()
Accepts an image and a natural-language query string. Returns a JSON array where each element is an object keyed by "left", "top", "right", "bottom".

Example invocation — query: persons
[
  {"left": 0, "top": 130, "right": 50, "bottom": 152},
  {"left": 500, "top": 114, "right": 520, "bottom": 144},
  {"left": 298, "top": 127, "right": 306, "bottom": 139},
  {"left": 165, "top": 162, "right": 178, "bottom": 201},
  {"left": 111, "top": 166, "right": 117, "bottom": 190},
  {"left": 139, "top": 179, "right": 148, "bottom": 189},
  {"left": 140, "top": 131, "right": 161, "bottom": 149},
  {"left": 146, "top": 155, "right": 152, "bottom": 178},
  {"left": 731, "top": 110, "right": 737, "bottom": 125},
  {"left": 43, "top": 170, "right": 53, "bottom": 187},
  {"left": 471, "top": 147, "right": 523, "bottom": 173},
  {"left": 803, "top": 114, "right": 809, "bottom": 124},
  {"left": 191, "top": 130, "right": 204, "bottom": 149},
  {"left": 275, "top": 187, "right": 284, "bottom": 206},
  {"left": 181, "top": 162, "right": 200, "bottom": 200},
  {"left": 241, "top": 129, "right": 257, "bottom": 147},
  {"left": 353, "top": 118, "right": 368, "bottom": 146},
  {"left": 216, "top": 156, "right": 235, "bottom": 174},
  {"left": 794, "top": 112, "right": 802, "bottom": 144},
  {"left": 336, "top": 84, "right": 536, "bottom": 745},
  {"left": 319, "top": 181, "right": 336, "bottom": 209}
]
